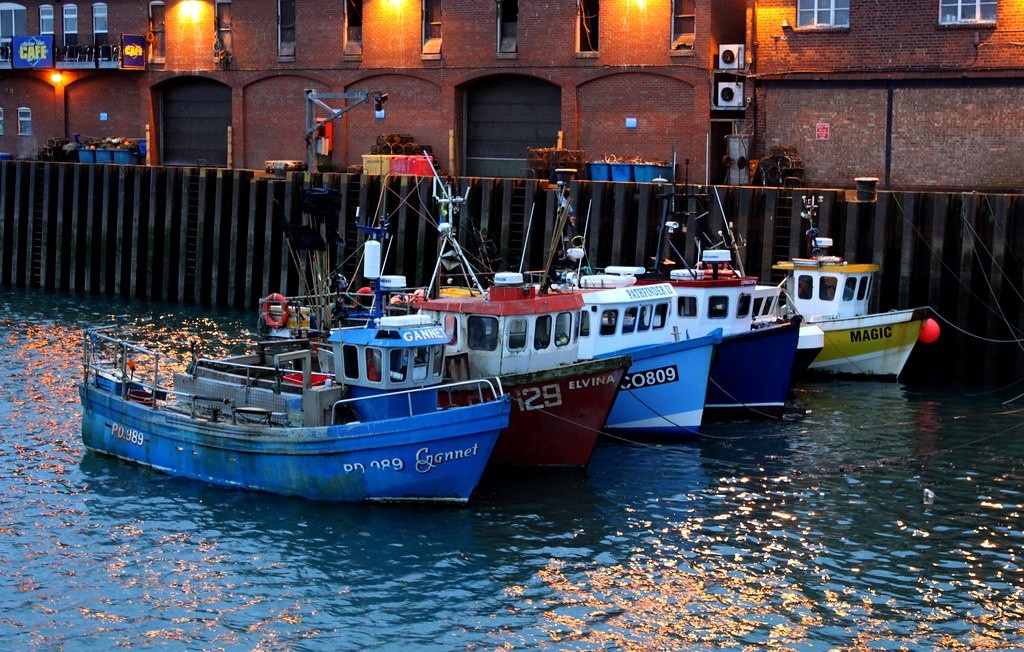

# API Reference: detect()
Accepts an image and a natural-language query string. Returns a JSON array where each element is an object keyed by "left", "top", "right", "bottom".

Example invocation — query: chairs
[{"left": 64, "top": 43, "right": 117, "bottom": 62}]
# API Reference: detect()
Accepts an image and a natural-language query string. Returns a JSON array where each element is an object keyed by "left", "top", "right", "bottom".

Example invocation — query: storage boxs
[
  {"left": 283, "top": 372, "right": 331, "bottom": 387},
  {"left": 93, "top": 372, "right": 143, "bottom": 396}
]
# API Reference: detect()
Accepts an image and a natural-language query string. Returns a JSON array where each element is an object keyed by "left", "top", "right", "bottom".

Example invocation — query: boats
[
  {"left": 77, "top": 208, "right": 514, "bottom": 509},
  {"left": 483, "top": 166, "right": 728, "bottom": 435},
  {"left": 751, "top": 281, "right": 825, "bottom": 388},
  {"left": 770, "top": 188, "right": 938, "bottom": 382},
  {"left": 593, "top": 152, "right": 805, "bottom": 425},
  {"left": 252, "top": 151, "right": 633, "bottom": 469}
]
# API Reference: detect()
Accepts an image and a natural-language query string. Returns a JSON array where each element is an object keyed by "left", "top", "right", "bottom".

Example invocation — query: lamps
[{"left": 374, "top": 92, "right": 389, "bottom": 119}]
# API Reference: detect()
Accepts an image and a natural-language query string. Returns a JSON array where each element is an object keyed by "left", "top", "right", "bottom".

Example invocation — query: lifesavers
[
  {"left": 670, "top": 269, "right": 705, "bottom": 281},
  {"left": 703, "top": 269, "right": 734, "bottom": 280},
  {"left": 353, "top": 286, "right": 377, "bottom": 322},
  {"left": 811, "top": 256, "right": 843, "bottom": 263},
  {"left": 263, "top": 293, "right": 290, "bottom": 329}
]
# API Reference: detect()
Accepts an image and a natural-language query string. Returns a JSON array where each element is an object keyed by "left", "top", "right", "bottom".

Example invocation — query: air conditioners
[
  {"left": 719, "top": 43, "right": 745, "bottom": 70},
  {"left": 717, "top": 82, "right": 745, "bottom": 107}
]
